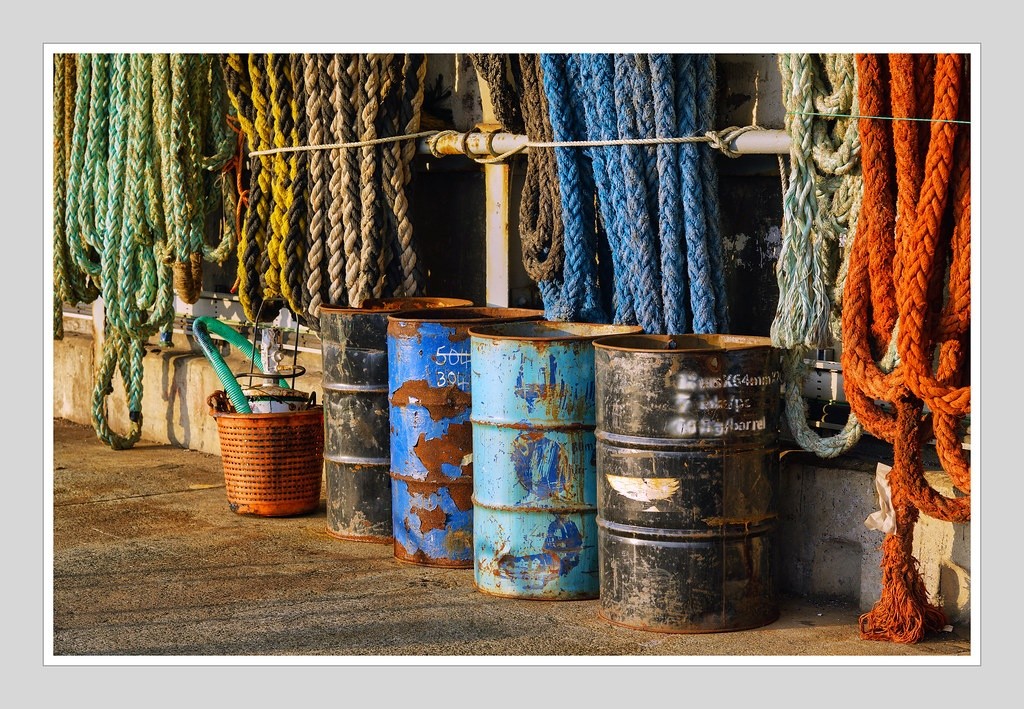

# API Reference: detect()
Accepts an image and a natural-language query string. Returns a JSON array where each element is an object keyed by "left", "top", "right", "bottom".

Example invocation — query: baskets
[{"left": 209, "top": 404, "right": 326, "bottom": 516}]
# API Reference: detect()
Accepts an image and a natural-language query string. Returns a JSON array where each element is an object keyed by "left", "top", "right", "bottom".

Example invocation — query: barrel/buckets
[
  {"left": 316, "top": 300, "right": 473, "bottom": 542},
  {"left": 385, "top": 310, "right": 544, "bottom": 567},
  {"left": 465, "top": 321, "right": 599, "bottom": 600},
  {"left": 589, "top": 336, "right": 775, "bottom": 635}
]
